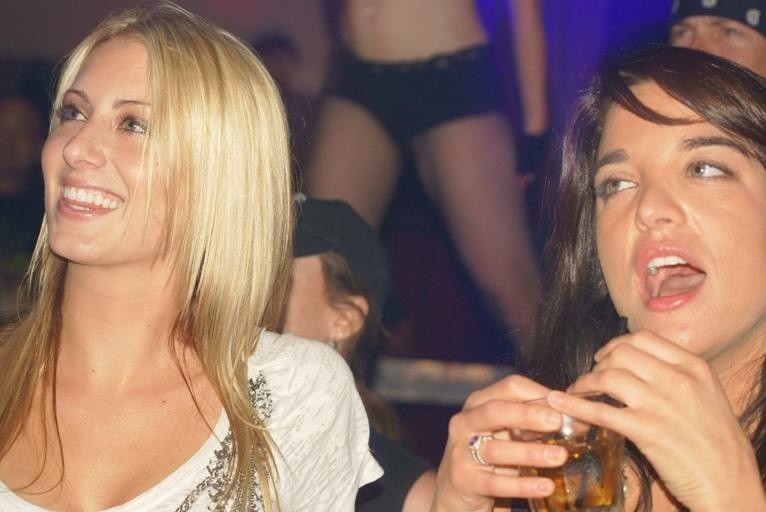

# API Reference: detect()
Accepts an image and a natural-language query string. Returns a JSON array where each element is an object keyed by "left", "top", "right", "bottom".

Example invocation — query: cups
[{"left": 507, "top": 390, "right": 653, "bottom": 512}]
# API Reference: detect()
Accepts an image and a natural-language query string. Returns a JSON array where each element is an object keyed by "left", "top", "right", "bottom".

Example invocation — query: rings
[{"left": 467, "top": 432, "right": 493, "bottom": 465}]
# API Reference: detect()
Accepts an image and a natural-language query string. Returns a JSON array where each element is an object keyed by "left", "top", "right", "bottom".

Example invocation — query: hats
[
  {"left": 291, "top": 196, "right": 387, "bottom": 308},
  {"left": 668, "top": 1, "right": 766, "bottom": 38}
]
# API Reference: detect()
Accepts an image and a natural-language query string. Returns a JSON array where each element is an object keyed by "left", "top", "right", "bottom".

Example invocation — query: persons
[
  {"left": 2, "top": 59, "right": 62, "bottom": 273},
  {"left": 668, "top": 0, "right": 765, "bottom": 81},
  {"left": 430, "top": 45, "right": 764, "bottom": 511},
  {"left": 288, "top": 0, "right": 549, "bottom": 374},
  {"left": 0, "top": 3, "right": 384, "bottom": 512},
  {"left": 281, "top": 196, "right": 438, "bottom": 512}
]
